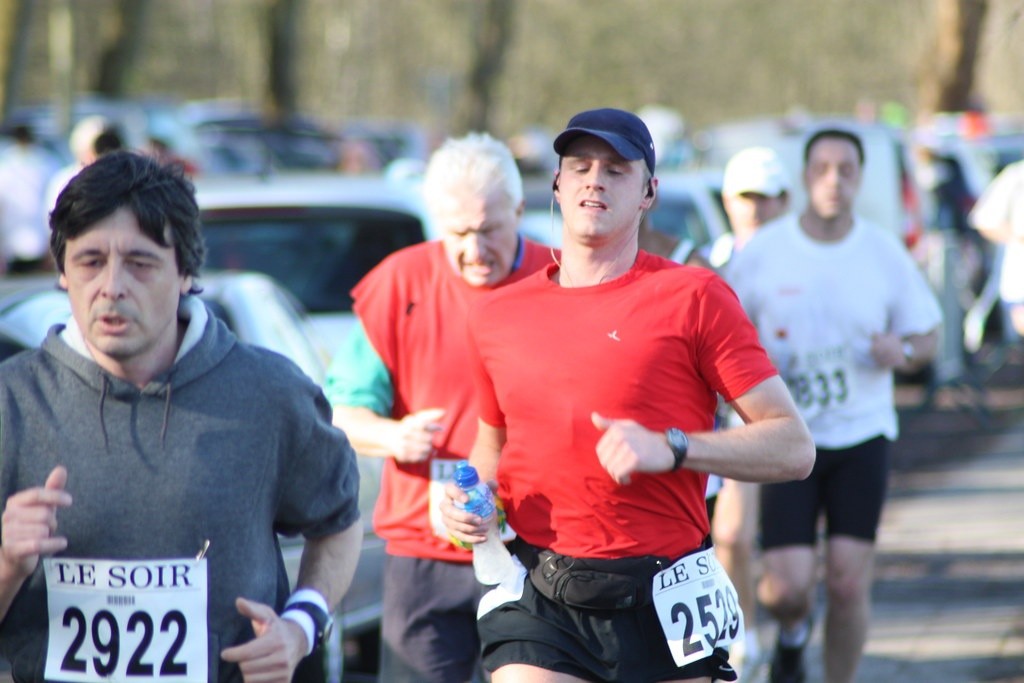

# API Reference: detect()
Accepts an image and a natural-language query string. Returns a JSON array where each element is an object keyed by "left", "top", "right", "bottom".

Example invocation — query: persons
[
  {"left": 441, "top": 107, "right": 815, "bottom": 683},
  {"left": 0, "top": 151, "right": 361, "bottom": 683},
  {"left": 319, "top": 129, "right": 561, "bottom": 683},
  {"left": 714, "top": 127, "right": 944, "bottom": 683},
  {"left": 0, "top": 116, "right": 125, "bottom": 274},
  {"left": 968, "top": 158, "right": 1024, "bottom": 339},
  {"left": 637, "top": 143, "right": 790, "bottom": 683}
]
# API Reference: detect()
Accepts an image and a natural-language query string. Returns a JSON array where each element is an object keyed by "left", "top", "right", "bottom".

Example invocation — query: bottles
[{"left": 454, "top": 460, "right": 512, "bottom": 585}]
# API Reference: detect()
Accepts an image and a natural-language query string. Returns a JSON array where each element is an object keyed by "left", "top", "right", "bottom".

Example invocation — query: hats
[
  {"left": 553, "top": 109, "right": 655, "bottom": 177},
  {"left": 722, "top": 148, "right": 788, "bottom": 198}
]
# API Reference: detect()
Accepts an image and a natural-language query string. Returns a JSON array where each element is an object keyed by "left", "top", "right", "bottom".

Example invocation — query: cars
[{"left": 0, "top": 97, "right": 1024, "bottom": 676}]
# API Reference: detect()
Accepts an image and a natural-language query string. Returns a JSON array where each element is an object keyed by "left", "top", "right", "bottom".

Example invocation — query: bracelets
[
  {"left": 280, "top": 586, "right": 332, "bottom": 656},
  {"left": 902, "top": 337, "right": 913, "bottom": 364}
]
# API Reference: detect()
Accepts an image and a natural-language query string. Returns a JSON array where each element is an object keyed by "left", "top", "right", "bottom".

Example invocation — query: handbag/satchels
[{"left": 509, "top": 537, "right": 706, "bottom": 607}]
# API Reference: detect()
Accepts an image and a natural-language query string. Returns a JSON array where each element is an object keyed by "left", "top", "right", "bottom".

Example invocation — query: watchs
[
  {"left": 283, "top": 602, "right": 333, "bottom": 650},
  {"left": 665, "top": 428, "right": 689, "bottom": 472}
]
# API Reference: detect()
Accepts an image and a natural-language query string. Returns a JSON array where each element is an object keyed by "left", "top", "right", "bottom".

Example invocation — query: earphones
[
  {"left": 552, "top": 172, "right": 560, "bottom": 191},
  {"left": 646, "top": 177, "right": 654, "bottom": 198}
]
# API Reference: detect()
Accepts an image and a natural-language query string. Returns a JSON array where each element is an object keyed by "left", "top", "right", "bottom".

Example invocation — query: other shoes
[
  {"left": 735, "top": 636, "right": 763, "bottom": 683},
  {"left": 768, "top": 614, "right": 813, "bottom": 683}
]
